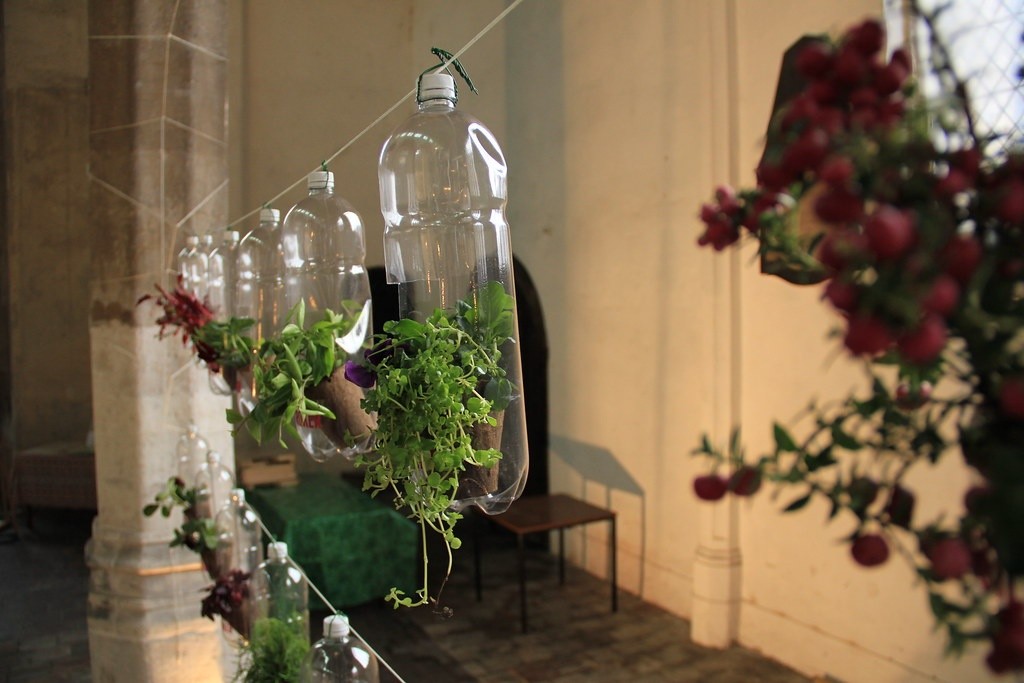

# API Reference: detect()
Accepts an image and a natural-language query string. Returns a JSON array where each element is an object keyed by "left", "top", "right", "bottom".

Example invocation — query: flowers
[
  {"left": 344, "top": 285, "right": 516, "bottom": 609},
  {"left": 226, "top": 295, "right": 361, "bottom": 446},
  {"left": 136, "top": 274, "right": 255, "bottom": 392},
  {"left": 682, "top": 0, "right": 1022, "bottom": 676},
  {"left": 143, "top": 477, "right": 208, "bottom": 516},
  {"left": 170, "top": 519, "right": 219, "bottom": 553},
  {"left": 196, "top": 571, "right": 253, "bottom": 621}
]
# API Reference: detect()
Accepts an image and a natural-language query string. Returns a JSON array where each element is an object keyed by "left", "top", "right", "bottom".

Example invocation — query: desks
[{"left": 253, "top": 468, "right": 419, "bottom": 612}]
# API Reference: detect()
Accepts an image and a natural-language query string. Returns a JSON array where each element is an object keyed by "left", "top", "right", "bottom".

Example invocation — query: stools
[{"left": 472, "top": 494, "right": 618, "bottom": 634}]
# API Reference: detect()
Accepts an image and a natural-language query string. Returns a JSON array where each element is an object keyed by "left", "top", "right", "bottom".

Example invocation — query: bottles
[
  {"left": 250, "top": 541, "right": 311, "bottom": 656},
  {"left": 177, "top": 235, "right": 195, "bottom": 297},
  {"left": 206, "top": 230, "right": 256, "bottom": 348},
  {"left": 238, "top": 207, "right": 284, "bottom": 425},
  {"left": 272, "top": 167, "right": 378, "bottom": 467},
  {"left": 374, "top": 74, "right": 529, "bottom": 519},
  {"left": 301, "top": 613, "right": 380, "bottom": 683},
  {"left": 212, "top": 487, "right": 264, "bottom": 583},
  {"left": 187, "top": 233, "right": 212, "bottom": 307},
  {"left": 177, "top": 424, "right": 209, "bottom": 489},
  {"left": 193, "top": 452, "right": 236, "bottom": 523}
]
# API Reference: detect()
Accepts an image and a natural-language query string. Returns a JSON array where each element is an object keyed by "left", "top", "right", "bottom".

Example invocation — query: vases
[
  {"left": 221, "top": 597, "right": 261, "bottom": 636},
  {"left": 302, "top": 359, "right": 375, "bottom": 450},
  {"left": 201, "top": 543, "right": 235, "bottom": 580},
  {"left": 447, "top": 409, "right": 506, "bottom": 499},
  {"left": 182, "top": 505, "right": 213, "bottom": 518}
]
